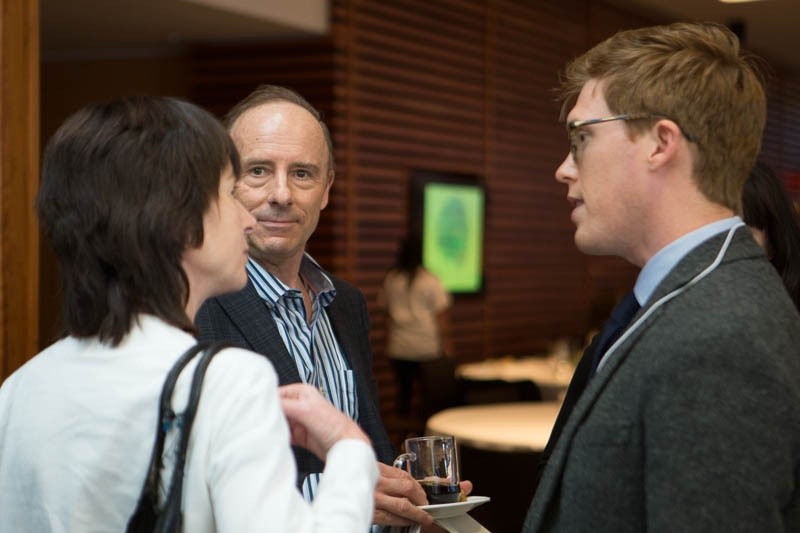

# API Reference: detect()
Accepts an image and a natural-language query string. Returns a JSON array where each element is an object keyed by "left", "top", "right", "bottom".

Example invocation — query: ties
[{"left": 543, "top": 291, "right": 642, "bottom": 477}]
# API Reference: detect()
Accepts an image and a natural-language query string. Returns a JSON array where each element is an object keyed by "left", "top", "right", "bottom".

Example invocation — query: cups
[{"left": 393, "top": 435, "right": 460, "bottom": 504}]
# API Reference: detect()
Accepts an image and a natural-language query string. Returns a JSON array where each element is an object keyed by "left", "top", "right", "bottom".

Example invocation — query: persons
[
  {"left": 0, "top": 95, "right": 380, "bottom": 533},
  {"left": 520, "top": 21, "right": 799, "bottom": 533},
  {"left": 192, "top": 83, "right": 475, "bottom": 533},
  {"left": 377, "top": 236, "right": 453, "bottom": 423}
]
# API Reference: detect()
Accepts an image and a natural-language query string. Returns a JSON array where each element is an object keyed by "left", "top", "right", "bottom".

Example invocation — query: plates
[{"left": 416, "top": 495, "right": 491, "bottom": 519}]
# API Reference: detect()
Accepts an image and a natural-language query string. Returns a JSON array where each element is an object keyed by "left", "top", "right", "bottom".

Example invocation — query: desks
[
  {"left": 460, "top": 354, "right": 574, "bottom": 397},
  {"left": 429, "top": 399, "right": 560, "bottom": 453}
]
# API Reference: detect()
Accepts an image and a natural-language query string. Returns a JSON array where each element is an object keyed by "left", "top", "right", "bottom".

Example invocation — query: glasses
[{"left": 566, "top": 113, "right": 694, "bottom": 162}]
